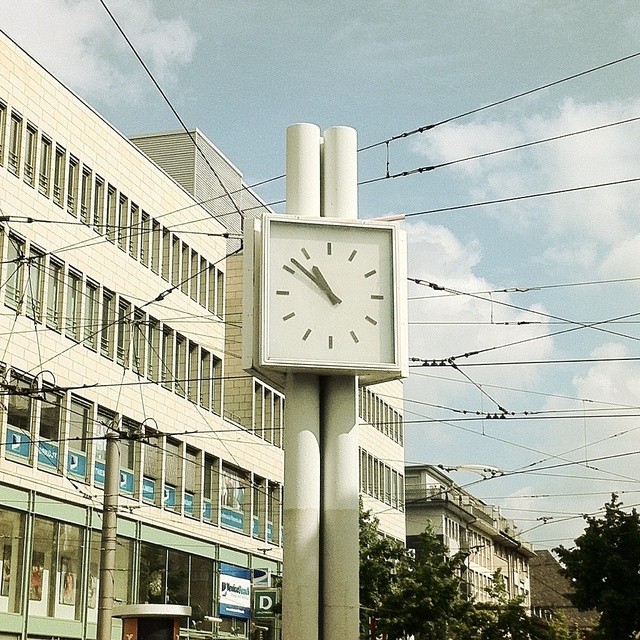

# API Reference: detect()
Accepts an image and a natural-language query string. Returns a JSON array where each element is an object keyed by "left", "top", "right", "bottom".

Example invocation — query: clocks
[{"left": 257, "top": 210, "right": 405, "bottom": 378}]
